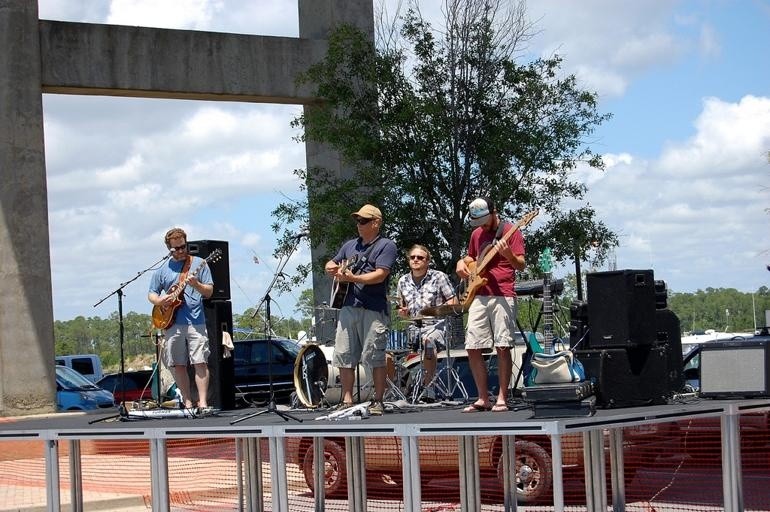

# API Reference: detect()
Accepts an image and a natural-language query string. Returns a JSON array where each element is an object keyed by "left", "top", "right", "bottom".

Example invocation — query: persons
[
  {"left": 395, "top": 245, "right": 458, "bottom": 405},
  {"left": 456, "top": 197, "right": 525, "bottom": 413},
  {"left": 325, "top": 204, "right": 397, "bottom": 415},
  {"left": 148, "top": 229, "right": 214, "bottom": 411}
]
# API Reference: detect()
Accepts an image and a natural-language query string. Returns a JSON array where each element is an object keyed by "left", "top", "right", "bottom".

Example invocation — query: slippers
[
  {"left": 492, "top": 403, "right": 507, "bottom": 412},
  {"left": 461, "top": 402, "right": 491, "bottom": 413}
]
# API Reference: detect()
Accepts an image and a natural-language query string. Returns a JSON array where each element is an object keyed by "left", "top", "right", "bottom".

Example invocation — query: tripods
[
  {"left": 353, "top": 370, "right": 406, "bottom": 403},
  {"left": 231, "top": 299, "right": 304, "bottom": 424},
  {"left": 414, "top": 316, "right": 469, "bottom": 404},
  {"left": 88, "top": 291, "right": 146, "bottom": 424},
  {"left": 136, "top": 338, "right": 177, "bottom": 410},
  {"left": 403, "top": 329, "right": 449, "bottom": 402}
]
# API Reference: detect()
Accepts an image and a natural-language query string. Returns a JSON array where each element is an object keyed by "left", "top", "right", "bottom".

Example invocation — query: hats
[
  {"left": 351, "top": 204, "right": 382, "bottom": 223},
  {"left": 468, "top": 196, "right": 494, "bottom": 227}
]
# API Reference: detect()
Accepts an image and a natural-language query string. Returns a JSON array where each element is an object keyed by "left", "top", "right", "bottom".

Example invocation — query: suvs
[{"left": 295, "top": 346, "right": 680, "bottom": 505}]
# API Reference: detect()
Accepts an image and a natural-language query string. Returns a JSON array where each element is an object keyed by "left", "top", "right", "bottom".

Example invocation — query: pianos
[{"left": 514, "top": 277, "right": 565, "bottom": 296}]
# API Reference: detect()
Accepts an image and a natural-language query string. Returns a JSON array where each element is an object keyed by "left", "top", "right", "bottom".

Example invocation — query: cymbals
[
  {"left": 303, "top": 304, "right": 341, "bottom": 311},
  {"left": 389, "top": 296, "right": 401, "bottom": 300},
  {"left": 400, "top": 315, "right": 435, "bottom": 323},
  {"left": 420, "top": 304, "right": 465, "bottom": 316}
]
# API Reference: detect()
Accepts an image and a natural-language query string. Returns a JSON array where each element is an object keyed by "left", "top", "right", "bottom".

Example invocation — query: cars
[
  {"left": 191, "top": 338, "right": 330, "bottom": 401},
  {"left": 49, "top": 365, "right": 116, "bottom": 410},
  {"left": 95, "top": 372, "right": 175, "bottom": 403},
  {"left": 289, "top": 431, "right": 648, "bottom": 500},
  {"left": 679, "top": 338, "right": 761, "bottom": 392}
]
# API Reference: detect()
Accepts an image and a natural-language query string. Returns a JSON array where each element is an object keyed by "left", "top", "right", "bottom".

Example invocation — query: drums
[
  {"left": 386, "top": 329, "right": 412, "bottom": 353},
  {"left": 293, "top": 342, "right": 373, "bottom": 408}
]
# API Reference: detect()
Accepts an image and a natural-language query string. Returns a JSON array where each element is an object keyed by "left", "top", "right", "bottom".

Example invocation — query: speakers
[
  {"left": 573, "top": 345, "right": 670, "bottom": 410},
  {"left": 180, "top": 300, "right": 238, "bottom": 412},
  {"left": 187, "top": 240, "right": 232, "bottom": 300},
  {"left": 585, "top": 268, "right": 659, "bottom": 348},
  {"left": 659, "top": 310, "right": 685, "bottom": 390}
]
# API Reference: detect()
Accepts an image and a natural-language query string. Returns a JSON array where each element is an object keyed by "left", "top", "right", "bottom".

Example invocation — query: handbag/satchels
[{"left": 529, "top": 351, "right": 586, "bottom": 384}]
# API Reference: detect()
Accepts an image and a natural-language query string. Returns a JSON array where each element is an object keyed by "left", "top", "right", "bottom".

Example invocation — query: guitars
[
  {"left": 151, "top": 333, "right": 176, "bottom": 401},
  {"left": 330, "top": 253, "right": 358, "bottom": 308},
  {"left": 152, "top": 248, "right": 222, "bottom": 330},
  {"left": 459, "top": 207, "right": 539, "bottom": 312},
  {"left": 528, "top": 247, "right": 555, "bottom": 386}
]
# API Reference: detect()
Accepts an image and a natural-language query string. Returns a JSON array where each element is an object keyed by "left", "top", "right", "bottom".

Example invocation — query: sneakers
[
  {"left": 329, "top": 400, "right": 352, "bottom": 413},
  {"left": 368, "top": 400, "right": 385, "bottom": 415},
  {"left": 422, "top": 389, "right": 436, "bottom": 400}
]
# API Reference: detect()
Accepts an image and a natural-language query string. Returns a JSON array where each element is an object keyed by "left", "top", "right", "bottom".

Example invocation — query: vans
[{"left": 48, "top": 354, "right": 106, "bottom": 394}]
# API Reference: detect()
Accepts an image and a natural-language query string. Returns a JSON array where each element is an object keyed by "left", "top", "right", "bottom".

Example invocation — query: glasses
[
  {"left": 174, "top": 243, "right": 188, "bottom": 251},
  {"left": 354, "top": 218, "right": 376, "bottom": 225},
  {"left": 411, "top": 256, "right": 426, "bottom": 260}
]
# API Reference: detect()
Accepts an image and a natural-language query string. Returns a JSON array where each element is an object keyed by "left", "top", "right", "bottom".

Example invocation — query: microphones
[
  {"left": 163, "top": 247, "right": 176, "bottom": 261},
  {"left": 291, "top": 230, "right": 310, "bottom": 240},
  {"left": 154, "top": 334, "right": 164, "bottom": 337}
]
